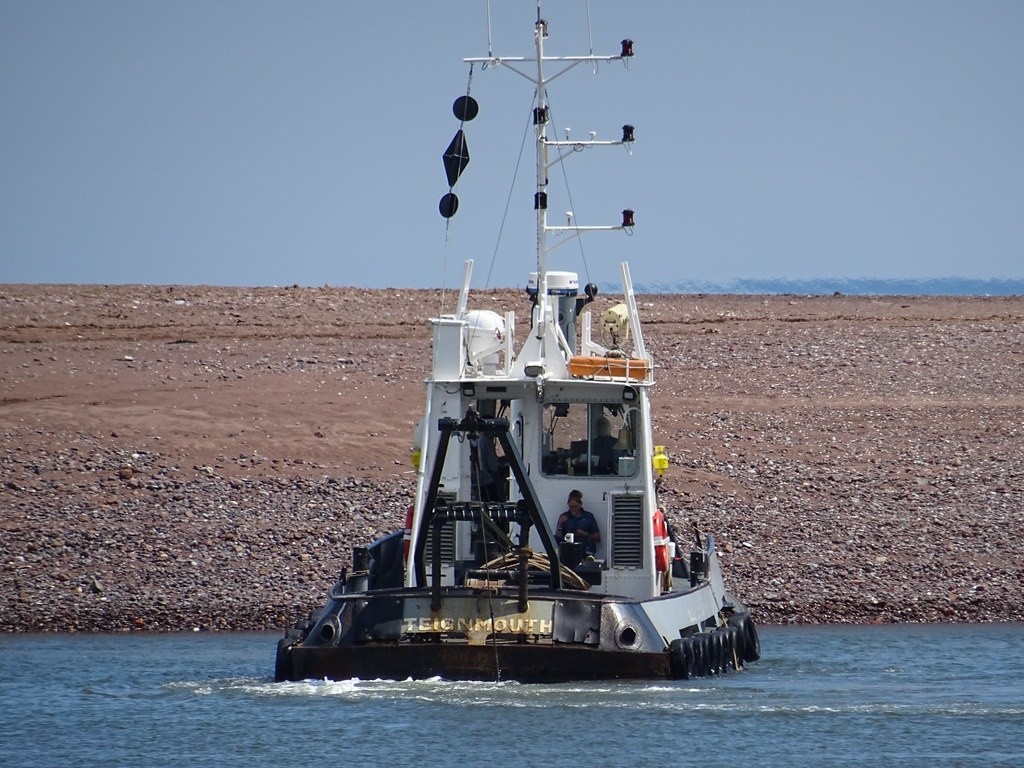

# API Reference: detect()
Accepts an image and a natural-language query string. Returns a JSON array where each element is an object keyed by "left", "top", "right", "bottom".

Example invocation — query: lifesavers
[
  {"left": 653, "top": 510, "right": 668, "bottom": 573},
  {"left": 670, "top": 611, "right": 761, "bottom": 680},
  {"left": 403, "top": 504, "right": 414, "bottom": 564}
]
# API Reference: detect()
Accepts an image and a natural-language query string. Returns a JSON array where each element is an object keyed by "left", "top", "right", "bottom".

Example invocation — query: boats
[{"left": 275, "top": 1, "right": 758, "bottom": 685}]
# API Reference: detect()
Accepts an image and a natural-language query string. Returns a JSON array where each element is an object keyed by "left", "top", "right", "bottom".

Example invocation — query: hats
[
  {"left": 568, "top": 490, "right": 582, "bottom": 505},
  {"left": 595, "top": 417, "right": 612, "bottom": 435}
]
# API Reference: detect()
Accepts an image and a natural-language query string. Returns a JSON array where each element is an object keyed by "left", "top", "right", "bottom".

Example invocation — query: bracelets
[{"left": 557, "top": 528, "right": 562, "bottom": 531}]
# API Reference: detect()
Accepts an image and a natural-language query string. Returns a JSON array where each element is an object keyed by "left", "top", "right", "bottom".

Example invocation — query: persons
[
  {"left": 573, "top": 417, "right": 632, "bottom": 475},
  {"left": 556, "top": 490, "right": 601, "bottom": 562},
  {"left": 472, "top": 415, "right": 502, "bottom": 501}
]
